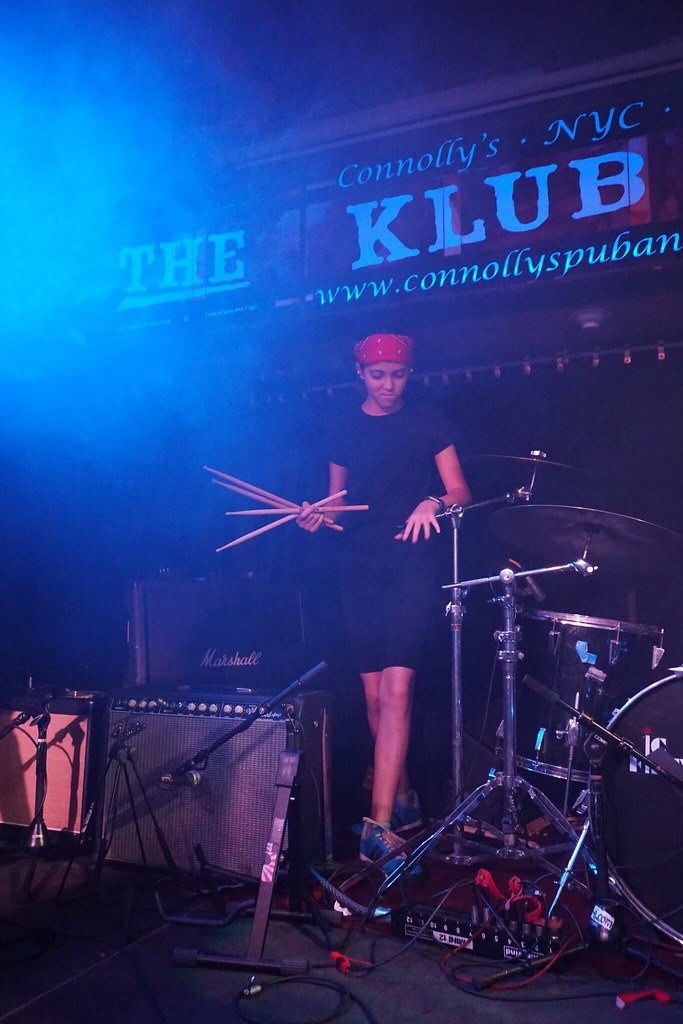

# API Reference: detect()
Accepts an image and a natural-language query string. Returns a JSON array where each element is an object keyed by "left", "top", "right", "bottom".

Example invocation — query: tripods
[
  {"left": 322, "top": 464, "right": 683, "bottom": 991},
  {"left": 0, "top": 687, "right": 185, "bottom": 908}
]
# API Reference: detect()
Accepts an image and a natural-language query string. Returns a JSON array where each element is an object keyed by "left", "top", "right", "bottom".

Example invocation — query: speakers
[
  {"left": 125, "top": 578, "right": 313, "bottom": 691},
  {"left": 98, "top": 695, "right": 335, "bottom": 894},
  {"left": 3, "top": 688, "right": 113, "bottom": 841}
]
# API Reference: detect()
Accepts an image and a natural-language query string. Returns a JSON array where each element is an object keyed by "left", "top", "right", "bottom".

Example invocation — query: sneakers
[
  {"left": 351, "top": 790, "right": 424, "bottom": 835},
  {"left": 359, "top": 817, "right": 424, "bottom": 877}
]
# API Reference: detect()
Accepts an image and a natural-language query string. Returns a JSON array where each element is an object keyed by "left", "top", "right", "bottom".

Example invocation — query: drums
[{"left": 480, "top": 601, "right": 683, "bottom": 943}]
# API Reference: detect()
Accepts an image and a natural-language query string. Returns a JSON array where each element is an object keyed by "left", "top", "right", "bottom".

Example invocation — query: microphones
[
  {"left": 155, "top": 771, "right": 201, "bottom": 787},
  {"left": 522, "top": 574, "right": 547, "bottom": 602}
]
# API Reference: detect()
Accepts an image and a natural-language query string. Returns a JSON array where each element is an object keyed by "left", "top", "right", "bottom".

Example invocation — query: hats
[{"left": 354, "top": 333, "right": 416, "bottom": 366}]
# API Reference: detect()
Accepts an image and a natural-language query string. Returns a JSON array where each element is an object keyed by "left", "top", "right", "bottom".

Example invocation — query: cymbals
[{"left": 473, "top": 449, "right": 683, "bottom": 577}]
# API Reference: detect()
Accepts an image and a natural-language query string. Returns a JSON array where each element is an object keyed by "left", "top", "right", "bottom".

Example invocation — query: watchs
[{"left": 422, "top": 495, "right": 445, "bottom": 515}]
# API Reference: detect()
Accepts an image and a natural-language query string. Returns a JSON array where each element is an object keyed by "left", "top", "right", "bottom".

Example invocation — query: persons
[{"left": 295, "top": 333, "right": 472, "bottom": 880}]
